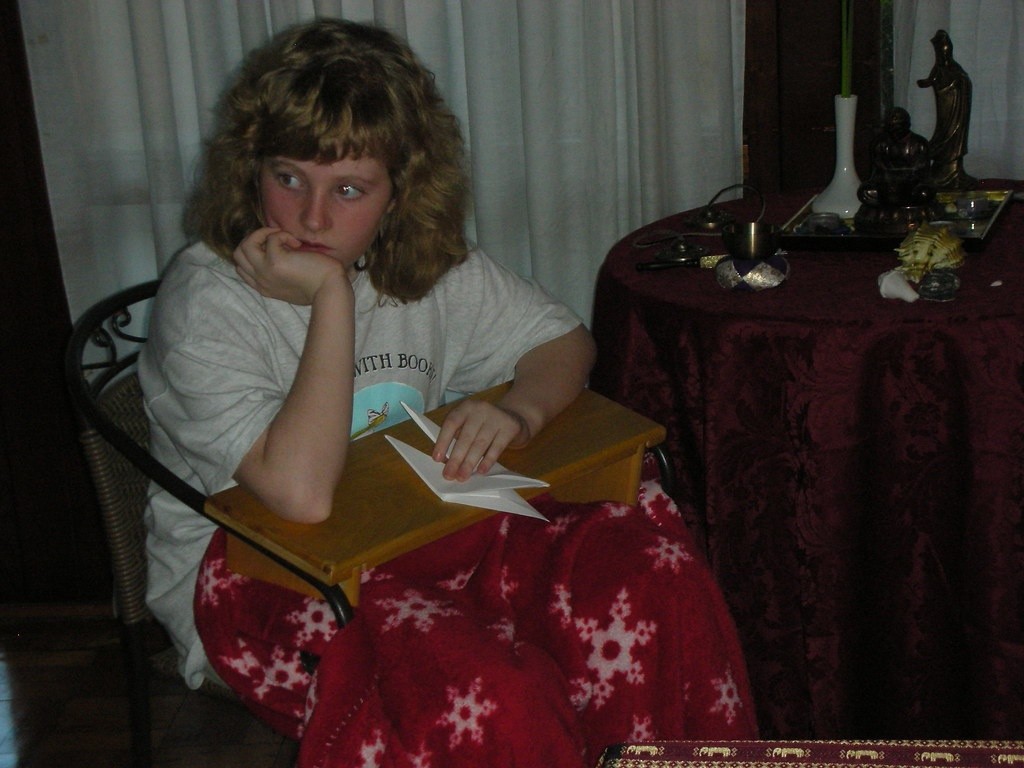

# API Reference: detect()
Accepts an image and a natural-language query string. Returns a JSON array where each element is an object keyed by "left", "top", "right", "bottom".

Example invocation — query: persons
[
  {"left": 877, "top": 29, "right": 977, "bottom": 193},
  {"left": 140, "top": 16, "right": 596, "bottom": 691}
]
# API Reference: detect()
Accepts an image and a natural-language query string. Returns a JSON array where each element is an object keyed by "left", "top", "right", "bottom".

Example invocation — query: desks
[
  {"left": 204, "top": 372, "right": 668, "bottom": 609},
  {"left": 586, "top": 180, "right": 1023, "bottom": 745}
]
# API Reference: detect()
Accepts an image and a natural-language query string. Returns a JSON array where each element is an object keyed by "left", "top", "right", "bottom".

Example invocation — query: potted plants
[{"left": 810, "top": 0, "right": 866, "bottom": 217}]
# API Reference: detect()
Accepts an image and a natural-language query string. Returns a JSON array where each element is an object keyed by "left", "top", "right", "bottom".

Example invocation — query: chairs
[{"left": 65, "top": 276, "right": 350, "bottom": 767}]
